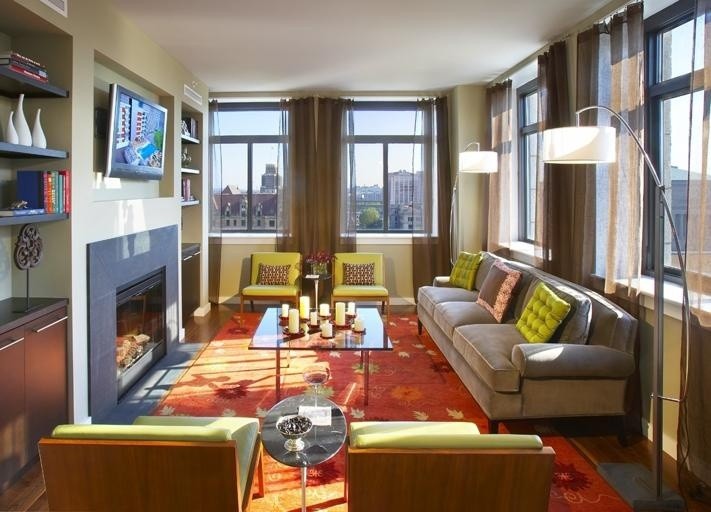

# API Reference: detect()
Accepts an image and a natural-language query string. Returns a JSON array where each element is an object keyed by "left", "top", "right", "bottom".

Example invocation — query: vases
[{"left": 311, "top": 264, "right": 327, "bottom": 275}]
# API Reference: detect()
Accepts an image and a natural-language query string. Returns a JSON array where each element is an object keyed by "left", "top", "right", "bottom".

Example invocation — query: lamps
[
  {"left": 446, "top": 140, "right": 498, "bottom": 266},
  {"left": 539, "top": 104, "right": 699, "bottom": 511}
]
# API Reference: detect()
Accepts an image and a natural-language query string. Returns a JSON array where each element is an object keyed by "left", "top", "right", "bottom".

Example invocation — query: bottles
[
  {"left": 6, "top": 93, "right": 47, "bottom": 150},
  {"left": 183, "top": 176, "right": 191, "bottom": 202}
]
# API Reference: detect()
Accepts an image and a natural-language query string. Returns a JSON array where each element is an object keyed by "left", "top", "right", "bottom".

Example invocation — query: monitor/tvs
[{"left": 103, "top": 83, "right": 169, "bottom": 179}]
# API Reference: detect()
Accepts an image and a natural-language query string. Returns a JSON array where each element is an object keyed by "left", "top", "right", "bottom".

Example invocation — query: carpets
[{"left": 147, "top": 311, "right": 634, "bottom": 512}]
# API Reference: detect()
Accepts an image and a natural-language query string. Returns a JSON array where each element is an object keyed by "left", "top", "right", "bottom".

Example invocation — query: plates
[{"left": 280, "top": 312, "right": 367, "bottom": 339}]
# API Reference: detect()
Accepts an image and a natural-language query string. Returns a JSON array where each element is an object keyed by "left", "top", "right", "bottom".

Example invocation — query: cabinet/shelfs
[
  {"left": 181, "top": 101, "right": 203, "bottom": 328},
  {"left": 0, "top": 296, "right": 68, "bottom": 495}
]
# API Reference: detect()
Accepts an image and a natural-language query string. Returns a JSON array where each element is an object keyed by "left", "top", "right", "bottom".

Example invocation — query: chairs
[
  {"left": 36, "top": 414, "right": 264, "bottom": 512},
  {"left": 331, "top": 252, "right": 390, "bottom": 329},
  {"left": 345, "top": 420, "right": 555, "bottom": 512},
  {"left": 238, "top": 251, "right": 303, "bottom": 328}
]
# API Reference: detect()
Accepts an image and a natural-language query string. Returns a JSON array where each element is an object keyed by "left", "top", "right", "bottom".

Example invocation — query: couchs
[{"left": 416, "top": 250, "right": 639, "bottom": 451}]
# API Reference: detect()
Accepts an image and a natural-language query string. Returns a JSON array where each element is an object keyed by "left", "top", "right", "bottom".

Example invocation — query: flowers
[{"left": 305, "top": 249, "right": 331, "bottom": 270}]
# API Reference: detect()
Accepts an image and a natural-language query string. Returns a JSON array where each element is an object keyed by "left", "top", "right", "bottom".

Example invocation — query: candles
[{"left": 279, "top": 295, "right": 365, "bottom": 339}]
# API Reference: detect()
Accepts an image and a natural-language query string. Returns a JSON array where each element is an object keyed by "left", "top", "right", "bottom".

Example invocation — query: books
[
  {"left": 182, "top": 176, "right": 195, "bottom": 202},
  {"left": 1, "top": 166, "right": 71, "bottom": 218},
  {"left": 0, "top": 51, "right": 48, "bottom": 82},
  {"left": 297, "top": 404, "right": 333, "bottom": 427}
]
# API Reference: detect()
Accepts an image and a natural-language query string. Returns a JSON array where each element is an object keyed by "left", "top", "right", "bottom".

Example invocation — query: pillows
[
  {"left": 255, "top": 262, "right": 291, "bottom": 286},
  {"left": 447, "top": 252, "right": 571, "bottom": 343},
  {"left": 342, "top": 261, "right": 376, "bottom": 286}
]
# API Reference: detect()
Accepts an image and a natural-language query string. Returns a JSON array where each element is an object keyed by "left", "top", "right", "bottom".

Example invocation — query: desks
[
  {"left": 304, "top": 271, "right": 331, "bottom": 310},
  {"left": 259, "top": 394, "right": 347, "bottom": 512}
]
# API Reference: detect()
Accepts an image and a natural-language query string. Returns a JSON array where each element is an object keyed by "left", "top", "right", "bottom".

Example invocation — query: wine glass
[{"left": 276, "top": 365, "right": 330, "bottom": 453}]
